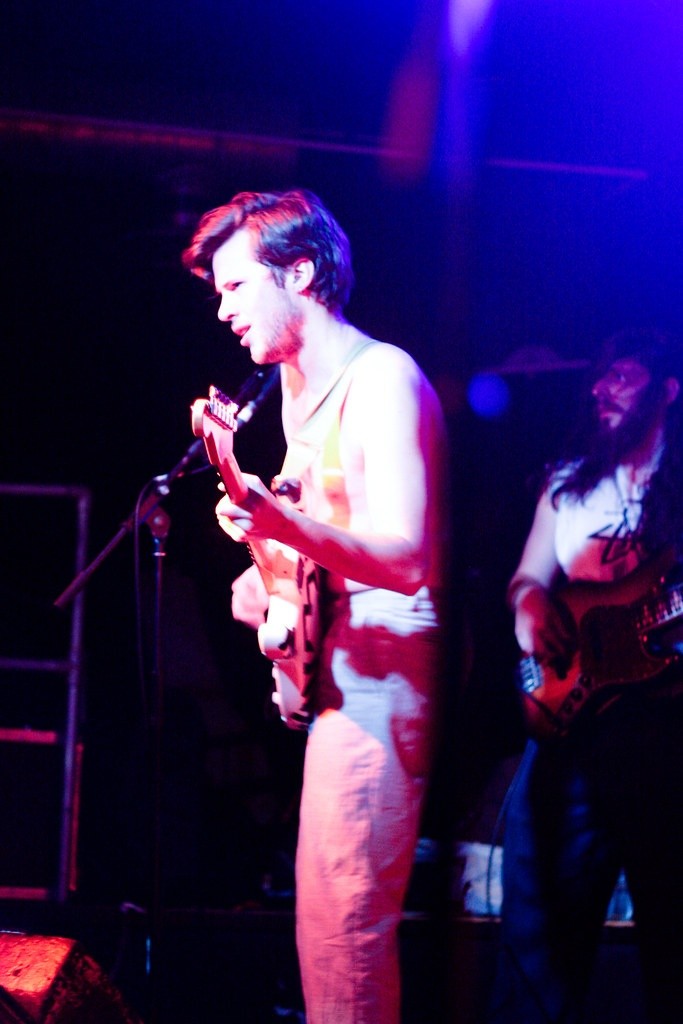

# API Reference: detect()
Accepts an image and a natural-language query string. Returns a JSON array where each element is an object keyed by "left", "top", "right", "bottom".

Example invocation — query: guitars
[
  {"left": 511, "top": 539, "right": 683, "bottom": 752},
  {"left": 189, "top": 385, "right": 324, "bottom": 733}
]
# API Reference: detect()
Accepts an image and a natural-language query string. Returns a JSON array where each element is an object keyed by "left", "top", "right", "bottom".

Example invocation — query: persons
[
  {"left": 497, "top": 320, "right": 683, "bottom": 1023},
  {"left": 182, "top": 185, "right": 448, "bottom": 1024}
]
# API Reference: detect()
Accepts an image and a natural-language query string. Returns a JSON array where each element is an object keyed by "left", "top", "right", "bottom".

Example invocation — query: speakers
[{"left": 0, "top": 931, "right": 143, "bottom": 1024}]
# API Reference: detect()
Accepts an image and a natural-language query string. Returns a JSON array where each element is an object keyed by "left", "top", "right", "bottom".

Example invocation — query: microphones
[{"left": 235, "top": 368, "right": 280, "bottom": 431}]
